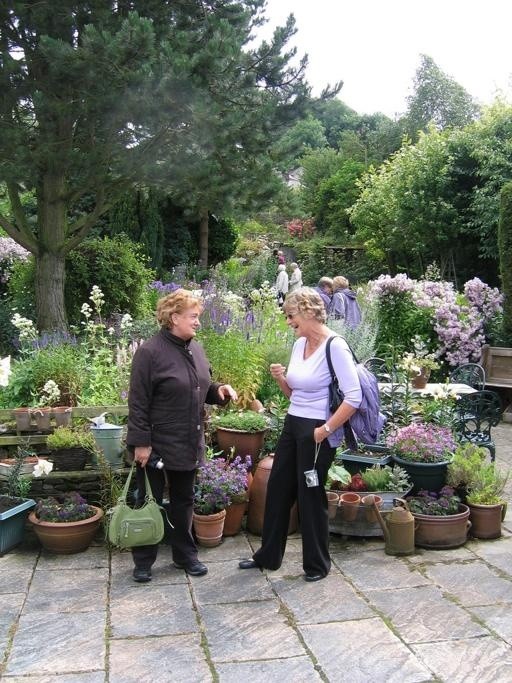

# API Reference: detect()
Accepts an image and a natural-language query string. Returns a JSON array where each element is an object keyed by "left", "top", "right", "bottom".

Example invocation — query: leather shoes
[
  {"left": 305, "top": 573, "right": 324, "bottom": 582},
  {"left": 239, "top": 558, "right": 260, "bottom": 569}
]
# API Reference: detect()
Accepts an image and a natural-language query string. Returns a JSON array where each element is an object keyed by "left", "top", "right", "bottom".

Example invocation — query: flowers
[
  {"left": 405, "top": 485, "right": 461, "bottom": 516},
  {"left": 396, "top": 335, "right": 444, "bottom": 377},
  {"left": 206, "top": 409, "right": 272, "bottom": 433},
  {"left": 33, "top": 490, "right": 91, "bottom": 521},
  {"left": 190, "top": 452, "right": 253, "bottom": 515},
  {"left": 32, "top": 379, "right": 61, "bottom": 410},
  {"left": 387, "top": 422, "right": 457, "bottom": 462}
]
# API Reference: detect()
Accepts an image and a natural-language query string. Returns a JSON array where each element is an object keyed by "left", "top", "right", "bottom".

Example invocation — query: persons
[
  {"left": 288, "top": 262, "right": 303, "bottom": 292},
  {"left": 316, "top": 276, "right": 335, "bottom": 317},
  {"left": 278, "top": 251, "right": 286, "bottom": 264},
  {"left": 333, "top": 276, "right": 362, "bottom": 332},
  {"left": 125, "top": 288, "right": 237, "bottom": 581},
  {"left": 239, "top": 285, "right": 362, "bottom": 581},
  {"left": 275, "top": 264, "right": 288, "bottom": 311}
]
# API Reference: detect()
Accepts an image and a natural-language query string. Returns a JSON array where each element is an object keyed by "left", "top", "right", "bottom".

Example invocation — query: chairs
[
  {"left": 363, "top": 357, "right": 397, "bottom": 382},
  {"left": 449, "top": 363, "right": 486, "bottom": 430},
  {"left": 450, "top": 391, "right": 503, "bottom": 462}
]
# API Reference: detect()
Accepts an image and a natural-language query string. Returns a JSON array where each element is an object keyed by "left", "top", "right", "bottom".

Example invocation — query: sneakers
[
  {"left": 174, "top": 559, "right": 208, "bottom": 576},
  {"left": 133, "top": 566, "right": 152, "bottom": 583}
]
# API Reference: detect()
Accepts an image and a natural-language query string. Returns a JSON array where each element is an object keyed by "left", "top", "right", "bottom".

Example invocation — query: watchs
[{"left": 324, "top": 423, "right": 333, "bottom": 434}]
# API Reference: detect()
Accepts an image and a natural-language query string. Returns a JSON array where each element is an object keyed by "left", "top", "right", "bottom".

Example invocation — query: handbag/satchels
[
  {"left": 107, "top": 501, "right": 165, "bottom": 549},
  {"left": 328, "top": 381, "right": 345, "bottom": 414}
]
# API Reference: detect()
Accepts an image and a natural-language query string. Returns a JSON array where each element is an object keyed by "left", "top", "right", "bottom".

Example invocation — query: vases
[
  {"left": 191, "top": 500, "right": 249, "bottom": 548},
  {"left": 410, "top": 504, "right": 472, "bottom": 549},
  {"left": 325, "top": 492, "right": 383, "bottom": 520},
  {"left": 406, "top": 366, "right": 431, "bottom": 388},
  {"left": 13, "top": 406, "right": 72, "bottom": 434},
  {"left": 216, "top": 425, "right": 265, "bottom": 465},
  {"left": 390, "top": 453, "right": 452, "bottom": 495},
  {"left": 334, "top": 442, "right": 392, "bottom": 476},
  {"left": 29, "top": 505, "right": 104, "bottom": 554}
]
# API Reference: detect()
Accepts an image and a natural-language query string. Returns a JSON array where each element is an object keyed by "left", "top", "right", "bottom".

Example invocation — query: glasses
[{"left": 285, "top": 311, "right": 302, "bottom": 320}]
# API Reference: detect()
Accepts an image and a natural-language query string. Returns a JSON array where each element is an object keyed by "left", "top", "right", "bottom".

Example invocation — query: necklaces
[{"left": 307, "top": 329, "right": 323, "bottom": 352}]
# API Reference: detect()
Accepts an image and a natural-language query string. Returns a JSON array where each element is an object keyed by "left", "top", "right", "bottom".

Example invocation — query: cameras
[{"left": 303, "top": 470, "right": 320, "bottom": 488}]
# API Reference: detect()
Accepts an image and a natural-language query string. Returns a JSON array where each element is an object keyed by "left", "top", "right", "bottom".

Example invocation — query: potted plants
[
  {"left": 0, "top": 433, "right": 36, "bottom": 552},
  {"left": 446, "top": 443, "right": 507, "bottom": 540},
  {"left": 45, "top": 424, "right": 97, "bottom": 471}
]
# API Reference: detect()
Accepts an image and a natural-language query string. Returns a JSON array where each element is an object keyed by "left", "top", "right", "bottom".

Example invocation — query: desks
[{"left": 377, "top": 383, "right": 480, "bottom": 394}]
